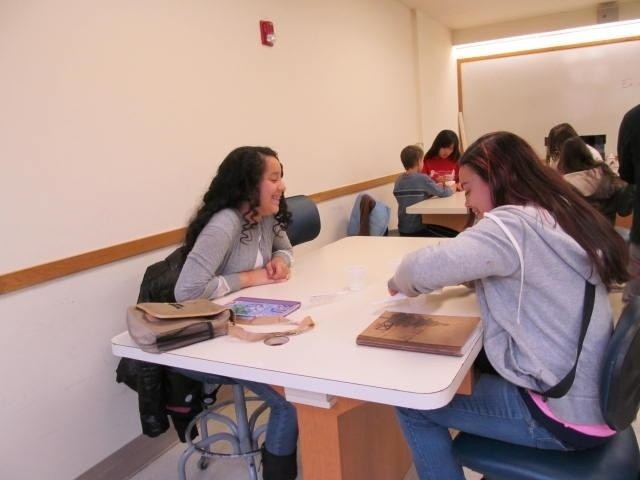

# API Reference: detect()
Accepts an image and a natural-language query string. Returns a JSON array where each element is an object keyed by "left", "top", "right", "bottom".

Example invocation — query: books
[
  {"left": 224, "top": 296, "right": 301, "bottom": 321},
  {"left": 357, "top": 307, "right": 483, "bottom": 357}
]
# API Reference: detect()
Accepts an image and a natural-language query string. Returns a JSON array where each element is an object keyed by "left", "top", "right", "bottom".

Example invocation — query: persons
[
  {"left": 422, "top": 129, "right": 463, "bottom": 194},
  {"left": 545, "top": 123, "right": 605, "bottom": 174},
  {"left": 558, "top": 137, "right": 637, "bottom": 235},
  {"left": 170, "top": 142, "right": 297, "bottom": 480},
  {"left": 382, "top": 131, "right": 630, "bottom": 480},
  {"left": 392, "top": 144, "right": 461, "bottom": 239},
  {"left": 616, "top": 103, "right": 640, "bottom": 303}
]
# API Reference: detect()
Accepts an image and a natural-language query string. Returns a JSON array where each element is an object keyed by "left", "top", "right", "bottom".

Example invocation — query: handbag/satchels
[{"left": 126, "top": 298, "right": 236, "bottom": 355}]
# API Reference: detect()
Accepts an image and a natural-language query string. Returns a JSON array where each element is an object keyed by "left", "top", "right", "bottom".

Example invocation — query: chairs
[
  {"left": 280, "top": 196, "right": 321, "bottom": 246},
  {"left": 116, "top": 247, "right": 271, "bottom": 480},
  {"left": 447, "top": 296, "right": 640, "bottom": 479}
]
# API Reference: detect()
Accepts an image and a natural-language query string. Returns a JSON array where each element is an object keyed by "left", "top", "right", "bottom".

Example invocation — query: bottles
[{"left": 607, "top": 152, "right": 615, "bottom": 168}]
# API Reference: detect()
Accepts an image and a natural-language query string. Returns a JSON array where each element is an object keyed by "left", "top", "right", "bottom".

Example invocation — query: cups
[{"left": 444, "top": 180, "right": 456, "bottom": 194}]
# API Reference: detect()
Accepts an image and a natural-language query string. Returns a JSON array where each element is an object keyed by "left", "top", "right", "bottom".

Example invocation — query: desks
[
  {"left": 111, "top": 236, "right": 482, "bottom": 480},
  {"left": 405, "top": 192, "right": 475, "bottom": 232}
]
